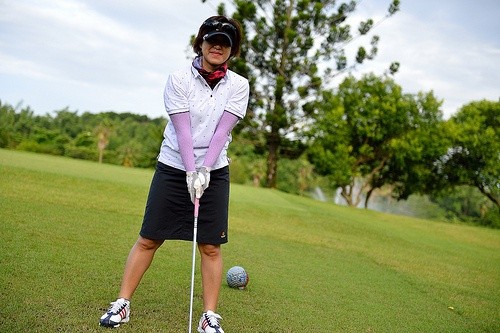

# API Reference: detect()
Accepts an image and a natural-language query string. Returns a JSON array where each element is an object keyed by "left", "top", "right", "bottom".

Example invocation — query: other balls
[{"left": 225, "top": 264, "right": 249, "bottom": 291}]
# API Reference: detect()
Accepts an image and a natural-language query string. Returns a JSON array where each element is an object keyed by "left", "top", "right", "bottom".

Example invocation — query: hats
[{"left": 203, "top": 19, "right": 237, "bottom": 46}]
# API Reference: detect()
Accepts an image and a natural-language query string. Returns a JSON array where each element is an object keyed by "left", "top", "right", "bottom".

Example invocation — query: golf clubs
[{"left": 187, "top": 189, "right": 202, "bottom": 333}]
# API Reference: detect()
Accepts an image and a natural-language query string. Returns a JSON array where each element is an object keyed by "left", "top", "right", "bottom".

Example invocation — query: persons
[{"left": 99, "top": 16, "right": 250, "bottom": 333}]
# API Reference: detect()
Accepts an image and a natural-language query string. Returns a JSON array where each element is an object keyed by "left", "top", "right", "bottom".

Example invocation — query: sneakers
[
  {"left": 98, "top": 297, "right": 131, "bottom": 326},
  {"left": 197, "top": 310, "right": 225, "bottom": 333}
]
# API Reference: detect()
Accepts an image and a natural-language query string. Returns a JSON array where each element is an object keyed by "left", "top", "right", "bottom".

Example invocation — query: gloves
[
  {"left": 198, "top": 166, "right": 211, "bottom": 191},
  {"left": 186, "top": 170, "right": 203, "bottom": 206}
]
